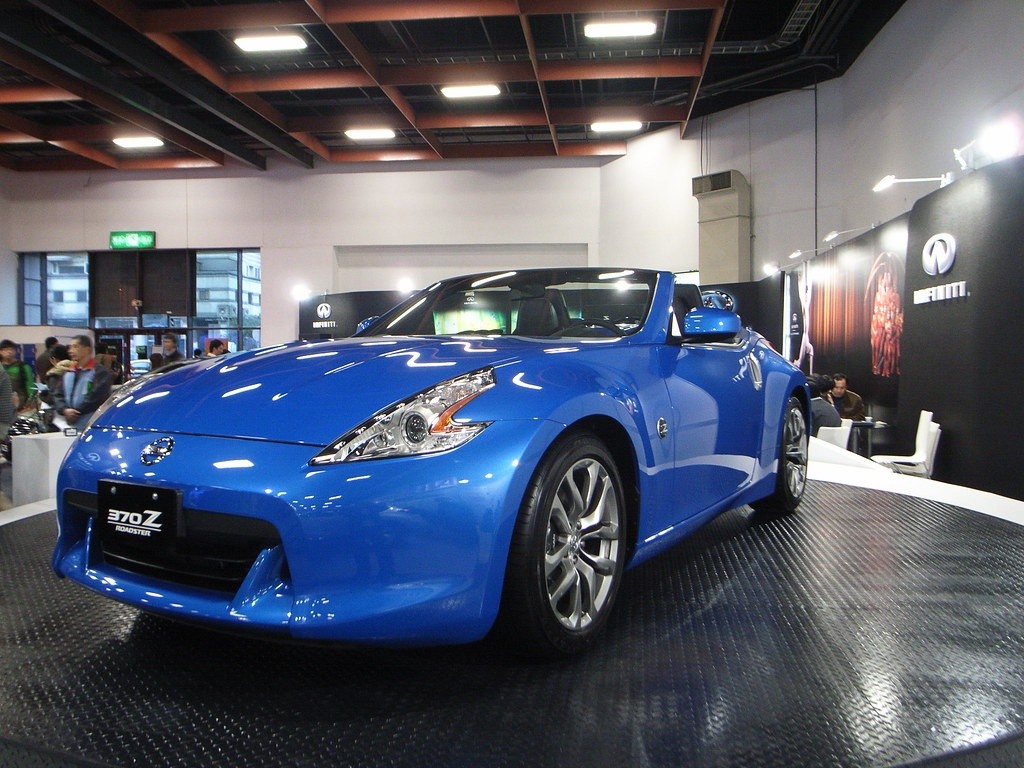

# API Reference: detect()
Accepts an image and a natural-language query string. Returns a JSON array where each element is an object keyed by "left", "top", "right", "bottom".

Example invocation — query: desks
[
  {"left": 11, "top": 429, "right": 81, "bottom": 506},
  {"left": 852, "top": 422, "right": 876, "bottom": 460}
]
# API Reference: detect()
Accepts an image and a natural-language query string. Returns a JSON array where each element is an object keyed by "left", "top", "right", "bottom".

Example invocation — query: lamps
[
  {"left": 873, "top": 170, "right": 953, "bottom": 194},
  {"left": 769, "top": 259, "right": 802, "bottom": 273},
  {"left": 948, "top": 138, "right": 984, "bottom": 176},
  {"left": 786, "top": 244, "right": 832, "bottom": 259},
  {"left": 823, "top": 220, "right": 879, "bottom": 242}
]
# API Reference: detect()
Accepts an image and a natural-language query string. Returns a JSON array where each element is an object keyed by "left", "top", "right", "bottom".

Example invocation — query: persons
[
  {"left": 94, "top": 342, "right": 123, "bottom": 385},
  {"left": 35, "top": 337, "right": 58, "bottom": 384},
  {"left": 53, "top": 335, "right": 112, "bottom": 432},
  {"left": 831, "top": 373, "right": 864, "bottom": 421},
  {"left": 150, "top": 333, "right": 184, "bottom": 371},
  {"left": 806, "top": 375, "right": 842, "bottom": 437},
  {"left": 194, "top": 349, "right": 202, "bottom": 358},
  {"left": 206, "top": 339, "right": 231, "bottom": 357},
  {"left": 0, "top": 339, "right": 33, "bottom": 440},
  {"left": 45, "top": 344, "right": 72, "bottom": 433}
]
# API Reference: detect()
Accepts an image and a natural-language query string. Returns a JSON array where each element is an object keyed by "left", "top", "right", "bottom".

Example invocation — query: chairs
[
  {"left": 869, "top": 409, "right": 942, "bottom": 480},
  {"left": 508, "top": 289, "right": 572, "bottom": 337},
  {"left": 670, "top": 284, "right": 703, "bottom": 339},
  {"left": 817, "top": 418, "right": 853, "bottom": 450}
]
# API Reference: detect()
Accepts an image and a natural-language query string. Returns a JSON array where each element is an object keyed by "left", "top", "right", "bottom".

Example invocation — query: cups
[{"left": 864, "top": 416, "right": 874, "bottom": 423}]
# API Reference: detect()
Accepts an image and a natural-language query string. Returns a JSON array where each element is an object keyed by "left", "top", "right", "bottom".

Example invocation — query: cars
[{"left": 46, "top": 264, "right": 814, "bottom": 657}]
